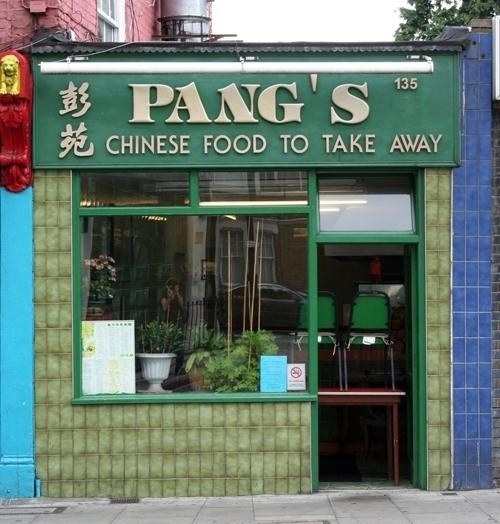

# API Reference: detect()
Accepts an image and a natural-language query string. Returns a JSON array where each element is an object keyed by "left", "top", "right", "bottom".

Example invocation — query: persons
[{"left": 160, "top": 278, "right": 185, "bottom": 331}]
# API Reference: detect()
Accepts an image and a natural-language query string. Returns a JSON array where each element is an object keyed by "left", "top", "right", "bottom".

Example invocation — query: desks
[{"left": 316, "top": 386, "right": 407, "bottom": 486}]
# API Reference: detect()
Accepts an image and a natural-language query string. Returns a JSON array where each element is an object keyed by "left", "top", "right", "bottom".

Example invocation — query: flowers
[{"left": 81, "top": 250, "right": 115, "bottom": 301}]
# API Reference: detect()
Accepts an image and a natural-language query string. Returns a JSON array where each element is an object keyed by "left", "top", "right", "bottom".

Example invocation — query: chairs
[
  {"left": 340, "top": 289, "right": 399, "bottom": 393},
  {"left": 285, "top": 289, "right": 343, "bottom": 392}
]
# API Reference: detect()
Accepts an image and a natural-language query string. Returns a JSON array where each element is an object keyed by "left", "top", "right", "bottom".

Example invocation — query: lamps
[
  {"left": 185, "top": 170, "right": 371, "bottom": 216},
  {"left": 35, "top": 53, "right": 437, "bottom": 77}
]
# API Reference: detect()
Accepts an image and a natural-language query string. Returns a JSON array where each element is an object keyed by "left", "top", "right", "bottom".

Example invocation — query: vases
[{"left": 83, "top": 295, "right": 111, "bottom": 319}]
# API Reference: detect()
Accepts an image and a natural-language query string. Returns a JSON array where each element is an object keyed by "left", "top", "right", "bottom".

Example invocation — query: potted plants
[
  {"left": 175, "top": 322, "right": 228, "bottom": 395},
  {"left": 128, "top": 319, "right": 186, "bottom": 393}
]
